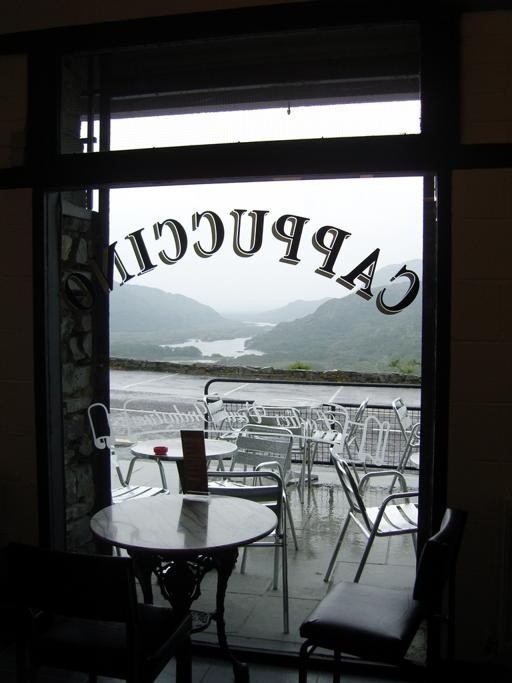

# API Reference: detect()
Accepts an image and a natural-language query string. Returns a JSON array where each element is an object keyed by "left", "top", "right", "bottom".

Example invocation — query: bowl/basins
[{"left": 152, "top": 445, "right": 168, "bottom": 455}]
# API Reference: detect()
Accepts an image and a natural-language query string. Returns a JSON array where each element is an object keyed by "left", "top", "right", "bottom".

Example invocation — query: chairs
[
  {"left": 110, "top": 384, "right": 423, "bottom": 584},
  {"left": 293, "top": 505, "right": 453, "bottom": 682},
  {"left": 12, "top": 544, "right": 134, "bottom": 603}
]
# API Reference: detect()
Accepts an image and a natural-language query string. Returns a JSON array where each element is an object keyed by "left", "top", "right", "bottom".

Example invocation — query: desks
[
  {"left": 91, "top": 493, "right": 280, "bottom": 663},
  {"left": 2, "top": 562, "right": 199, "bottom": 681}
]
